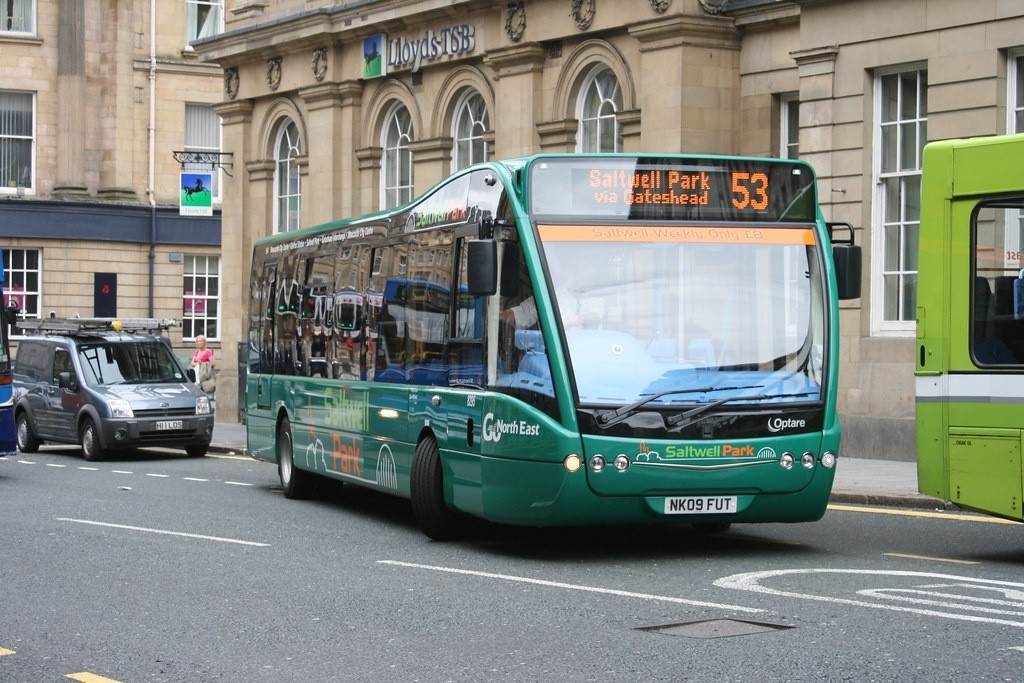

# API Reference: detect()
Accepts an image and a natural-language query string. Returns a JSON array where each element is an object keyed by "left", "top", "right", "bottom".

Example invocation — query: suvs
[{"left": 15, "top": 311, "right": 215, "bottom": 458}]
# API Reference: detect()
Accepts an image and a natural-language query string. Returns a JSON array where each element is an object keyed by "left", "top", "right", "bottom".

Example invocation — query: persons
[
  {"left": 497, "top": 266, "right": 596, "bottom": 370},
  {"left": 191, "top": 335, "right": 215, "bottom": 385}
]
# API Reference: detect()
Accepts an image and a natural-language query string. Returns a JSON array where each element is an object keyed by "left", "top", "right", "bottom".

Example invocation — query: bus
[
  {"left": 914, "top": 133, "right": 1023, "bottom": 525},
  {"left": 0, "top": 247, "right": 20, "bottom": 459},
  {"left": 245, "top": 152, "right": 863, "bottom": 540}
]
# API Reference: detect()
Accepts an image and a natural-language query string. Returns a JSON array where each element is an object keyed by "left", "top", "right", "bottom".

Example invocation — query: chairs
[
  {"left": 501, "top": 298, "right": 539, "bottom": 371},
  {"left": 974, "top": 275, "right": 1023, "bottom": 364}
]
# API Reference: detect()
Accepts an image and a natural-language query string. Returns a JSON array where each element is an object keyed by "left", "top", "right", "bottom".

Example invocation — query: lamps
[{"left": 17, "top": 187, "right": 25, "bottom": 197}]
